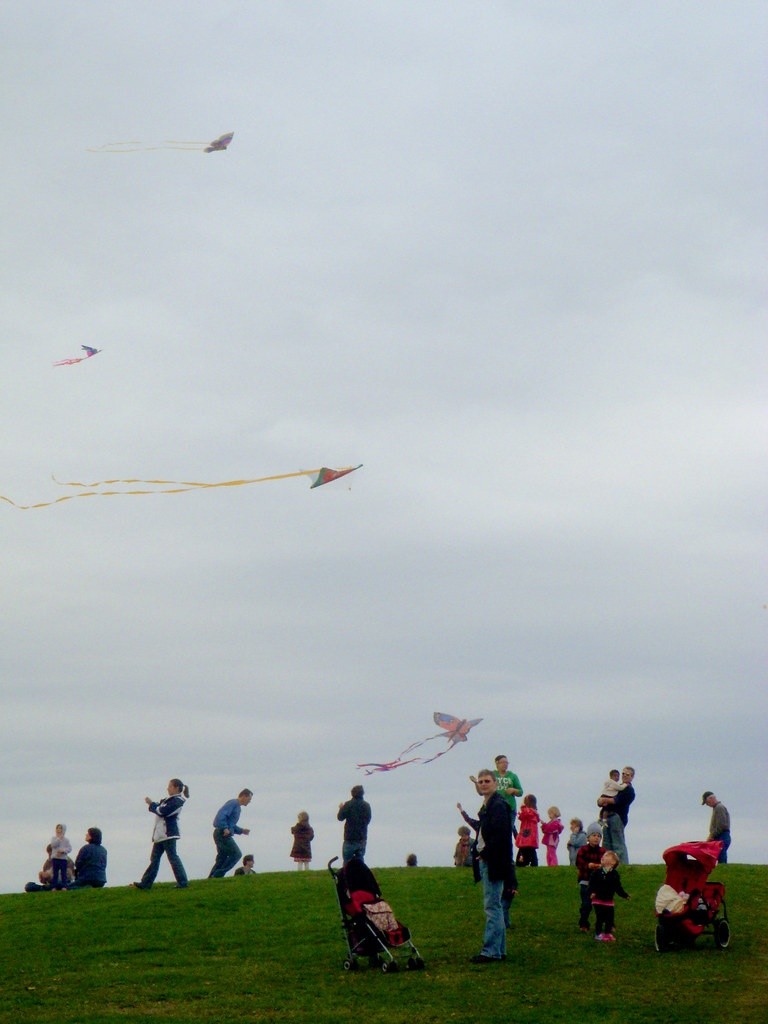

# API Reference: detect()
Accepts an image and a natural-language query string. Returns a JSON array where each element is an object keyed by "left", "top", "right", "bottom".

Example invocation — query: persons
[
  {"left": 235, "top": 855, "right": 256, "bottom": 876},
  {"left": 208, "top": 788, "right": 254, "bottom": 877},
  {"left": 290, "top": 811, "right": 314, "bottom": 871},
  {"left": 24, "top": 823, "right": 107, "bottom": 891},
  {"left": 133, "top": 779, "right": 190, "bottom": 891},
  {"left": 702, "top": 791, "right": 732, "bottom": 863},
  {"left": 454, "top": 755, "right": 635, "bottom": 962},
  {"left": 337, "top": 785, "right": 371, "bottom": 866}
]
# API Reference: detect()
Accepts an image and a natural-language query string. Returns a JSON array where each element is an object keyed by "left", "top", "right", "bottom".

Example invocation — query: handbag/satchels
[{"left": 363, "top": 901, "right": 398, "bottom": 931}]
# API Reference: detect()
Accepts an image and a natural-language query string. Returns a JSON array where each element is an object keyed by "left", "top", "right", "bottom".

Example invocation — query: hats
[
  {"left": 702, "top": 791, "right": 713, "bottom": 805},
  {"left": 587, "top": 823, "right": 602, "bottom": 838}
]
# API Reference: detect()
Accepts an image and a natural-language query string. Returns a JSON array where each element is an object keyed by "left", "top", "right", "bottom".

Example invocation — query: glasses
[
  {"left": 622, "top": 771, "right": 631, "bottom": 777},
  {"left": 478, "top": 780, "right": 490, "bottom": 784}
]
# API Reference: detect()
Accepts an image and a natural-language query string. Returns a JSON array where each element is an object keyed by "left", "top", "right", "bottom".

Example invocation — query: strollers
[
  {"left": 329, "top": 847, "right": 424, "bottom": 972},
  {"left": 655, "top": 840, "right": 730, "bottom": 952}
]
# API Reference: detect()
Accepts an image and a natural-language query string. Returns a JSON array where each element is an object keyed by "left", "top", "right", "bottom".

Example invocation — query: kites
[
  {"left": 0, "top": 463, "right": 365, "bottom": 509},
  {"left": 84, "top": 131, "right": 234, "bottom": 154},
  {"left": 52, "top": 345, "right": 104, "bottom": 367},
  {"left": 355, "top": 711, "right": 484, "bottom": 776}
]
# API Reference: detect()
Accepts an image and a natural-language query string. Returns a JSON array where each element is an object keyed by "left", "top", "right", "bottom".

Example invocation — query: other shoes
[
  {"left": 62, "top": 888, "right": 67, "bottom": 891},
  {"left": 606, "top": 934, "right": 616, "bottom": 941},
  {"left": 133, "top": 882, "right": 151, "bottom": 890},
  {"left": 470, "top": 955, "right": 501, "bottom": 963},
  {"left": 52, "top": 888, "right": 56, "bottom": 891},
  {"left": 595, "top": 934, "right": 610, "bottom": 941},
  {"left": 500, "top": 955, "right": 507, "bottom": 961}
]
